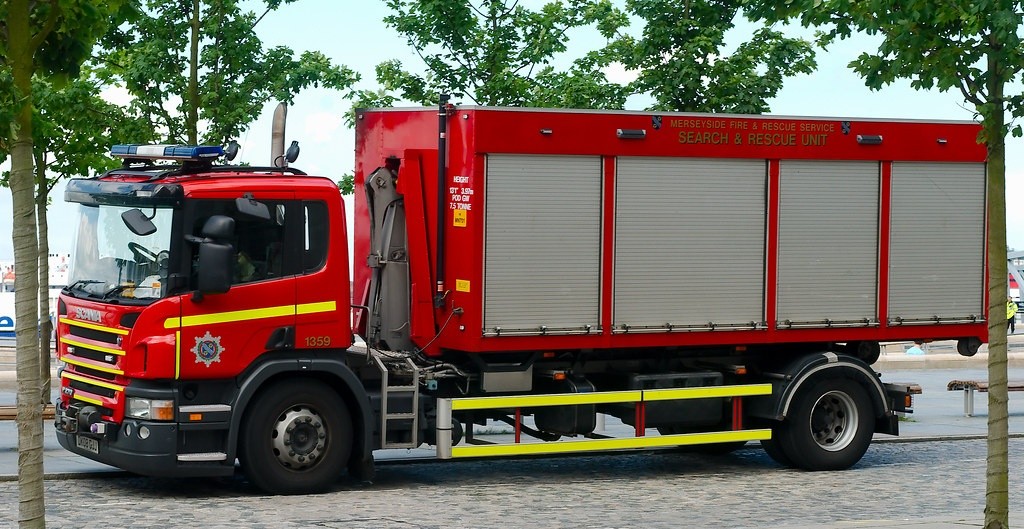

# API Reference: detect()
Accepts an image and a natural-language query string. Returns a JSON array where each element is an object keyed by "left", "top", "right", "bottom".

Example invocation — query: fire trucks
[{"left": 51, "top": 103, "right": 991, "bottom": 498}]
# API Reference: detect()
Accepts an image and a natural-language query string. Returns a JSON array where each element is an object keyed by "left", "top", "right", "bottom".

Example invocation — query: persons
[{"left": 1007, "top": 296, "right": 1018, "bottom": 334}]
